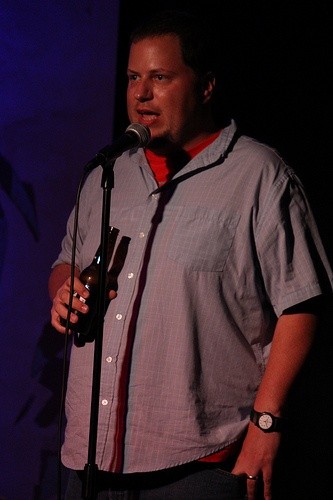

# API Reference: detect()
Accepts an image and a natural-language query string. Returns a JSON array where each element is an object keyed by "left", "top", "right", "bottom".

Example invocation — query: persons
[{"left": 46, "top": 9, "right": 332, "bottom": 500}]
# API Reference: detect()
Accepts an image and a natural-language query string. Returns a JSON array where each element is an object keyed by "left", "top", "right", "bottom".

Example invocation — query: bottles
[{"left": 61, "top": 225, "right": 121, "bottom": 336}]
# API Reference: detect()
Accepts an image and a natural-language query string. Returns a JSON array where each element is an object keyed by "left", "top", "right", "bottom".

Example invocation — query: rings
[{"left": 246, "top": 475, "right": 259, "bottom": 481}]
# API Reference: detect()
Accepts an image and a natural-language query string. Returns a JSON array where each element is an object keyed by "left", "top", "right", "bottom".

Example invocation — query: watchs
[{"left": 248, "top": 408, "right": 284, "bottom": 435}]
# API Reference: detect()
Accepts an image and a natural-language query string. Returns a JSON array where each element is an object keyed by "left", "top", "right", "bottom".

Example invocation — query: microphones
[{"left": 83, "top": 123, "right": 152, "bottom": 175}]
